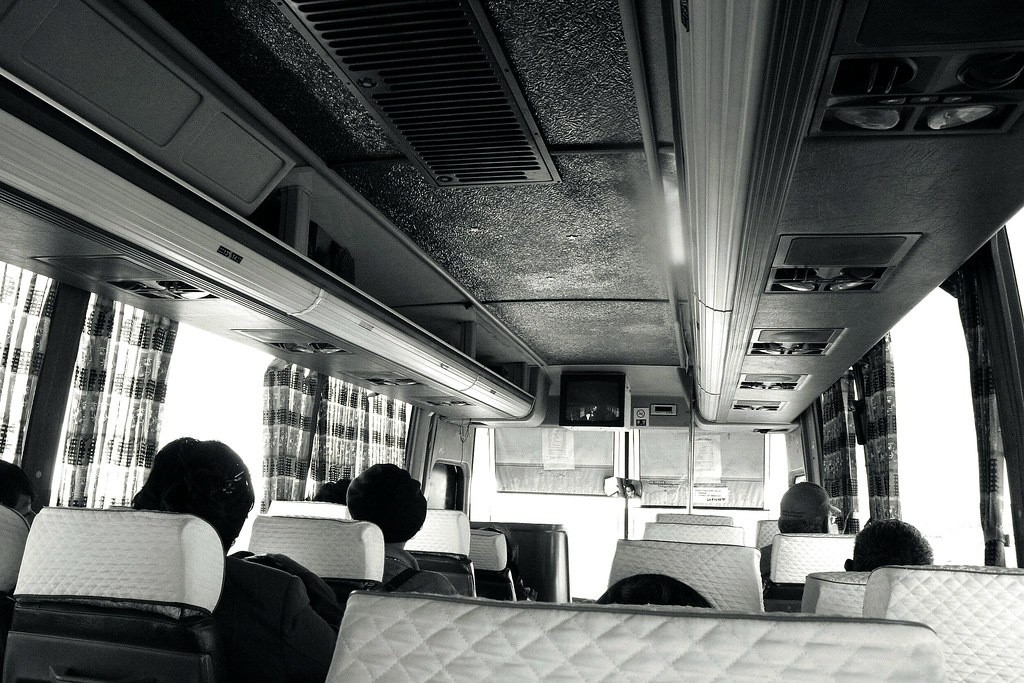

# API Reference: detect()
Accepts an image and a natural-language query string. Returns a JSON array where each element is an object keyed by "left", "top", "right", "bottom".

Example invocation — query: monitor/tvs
[{"left": 559, "top": 373, "right": 631, "bottom": 431}]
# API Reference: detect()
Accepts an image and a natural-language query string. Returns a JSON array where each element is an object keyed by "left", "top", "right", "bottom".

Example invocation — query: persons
[
  {"left": 127, "top": 438, "right": 336, "bottom": 683},
  {"left": 476, "top": 525, "right": 537, "bottom": 602},
  {"left": 778, "top": 482, "right": 843, "bottom": 534},
  {"left": 311, "top": 464, "right": 461, "bottom": 610},
  {"left": 844, "top": 520, "right": 931, "bottom": 572},
  {"left": 0, "top": 460, "right": 38, "bottom": 527}
]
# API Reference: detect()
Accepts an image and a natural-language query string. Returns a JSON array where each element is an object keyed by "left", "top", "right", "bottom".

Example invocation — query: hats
[
  {"left": 779, "top": 481, "right": 842, "bottom": 519},
  {"left": 347, "top": 463, "right": 426, "bottom": 542}
]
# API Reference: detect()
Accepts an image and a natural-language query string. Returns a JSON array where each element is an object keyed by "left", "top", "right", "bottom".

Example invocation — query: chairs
[{"left": 0, "top": 507, "right": 1024, "bottom": 683}]
